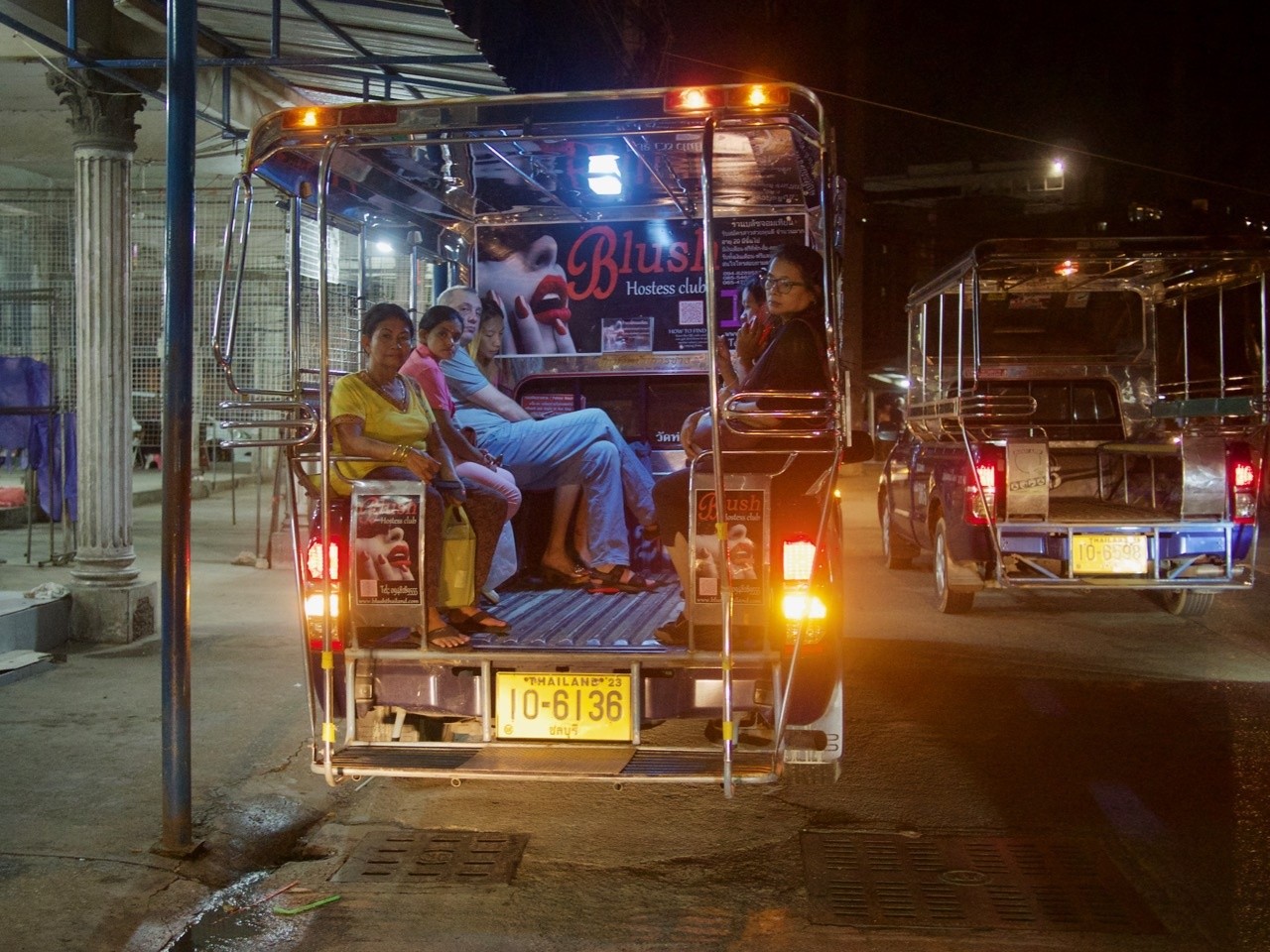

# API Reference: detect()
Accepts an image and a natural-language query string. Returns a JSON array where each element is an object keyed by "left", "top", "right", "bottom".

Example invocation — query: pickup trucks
[
  {"left": 211, "top": 75, "right": 876, "bottom": 782},
  {"left": 877, "top": 229, "right": 1270, "bottom": 615}
]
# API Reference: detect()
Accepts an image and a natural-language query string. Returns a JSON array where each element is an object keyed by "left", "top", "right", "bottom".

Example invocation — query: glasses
[{"left": 759, "top": 273, "right": 808, "bottom": 295}]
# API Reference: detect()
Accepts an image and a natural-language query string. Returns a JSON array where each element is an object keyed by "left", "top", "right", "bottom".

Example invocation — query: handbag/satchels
[
  {"left": 693, "top": 410, "right": 766, "bottom": 458},
  {"left": 441, "top": 498, "right": 476, "bottom": 608}
]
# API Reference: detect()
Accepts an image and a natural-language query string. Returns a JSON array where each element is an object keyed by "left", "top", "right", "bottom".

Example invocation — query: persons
[
  {"left": 328, "top": 303, "right": 511, "bottom": 652},
  {"left": 398, "top": 286, "right": 662, "bottom": 604},
  {"left": 604, "top": 319, "right": 628, "bottom": 350},
  {"left": 696, "top": 519, "right": 757, "bottom": 579},
  {"left": 477, "top": 226, "right": 577, "bottom": 355},
  {"left": 651, "top": 244, "right": 824, "bottom": 645},
  {"left": 357, "top": 515, "right": 415, "bottom": 581}
]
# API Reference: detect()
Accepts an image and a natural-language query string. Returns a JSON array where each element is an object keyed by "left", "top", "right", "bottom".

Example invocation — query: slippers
[
  {"left": 407, "top": 623, "right": 472, "bottom": 653},
  {"left": 452, "top": 610, "right": 510, "bottom": 633},
  {"left": 543, "top": 559, "right": 593, "bottom": 586}
]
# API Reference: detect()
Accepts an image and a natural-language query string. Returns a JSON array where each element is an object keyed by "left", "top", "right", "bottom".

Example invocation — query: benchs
[
  {"left": 688, "top": 388, "right": 843, "bottom": 499},
  {"left": 282, "top": 369, "right": 403, "bottom": 506},
  {"left": 907, "top": 396, "right": 1061, "bottom": 489},
  {"left": 1097, "top": 396, "right": 1262, "bottom": 511}
]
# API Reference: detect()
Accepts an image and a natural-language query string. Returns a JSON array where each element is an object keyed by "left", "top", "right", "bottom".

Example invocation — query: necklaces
[{"left": 364, "top": 370, "right": 407, "bottom": 413}]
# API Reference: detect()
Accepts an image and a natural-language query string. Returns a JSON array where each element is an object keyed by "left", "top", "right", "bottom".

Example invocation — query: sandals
[{"left": 585, "top": 563, "right": 659, "bottom": 593}]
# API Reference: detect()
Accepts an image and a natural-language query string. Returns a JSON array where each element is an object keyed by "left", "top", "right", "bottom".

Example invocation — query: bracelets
[
  {"left": 723, "top": 374, "right": 738, "bottom": 389},
  {"left": 391, "top": 443, "right": 413, "bottom": 461}
]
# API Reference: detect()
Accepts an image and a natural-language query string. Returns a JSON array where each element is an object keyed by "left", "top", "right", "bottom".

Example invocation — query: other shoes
[
  {"left": 638, "top": 523, "right": 673, "bottom": 565},
  {"left": 657, "top": 612, "right": 724, "bottom": 650}
]
